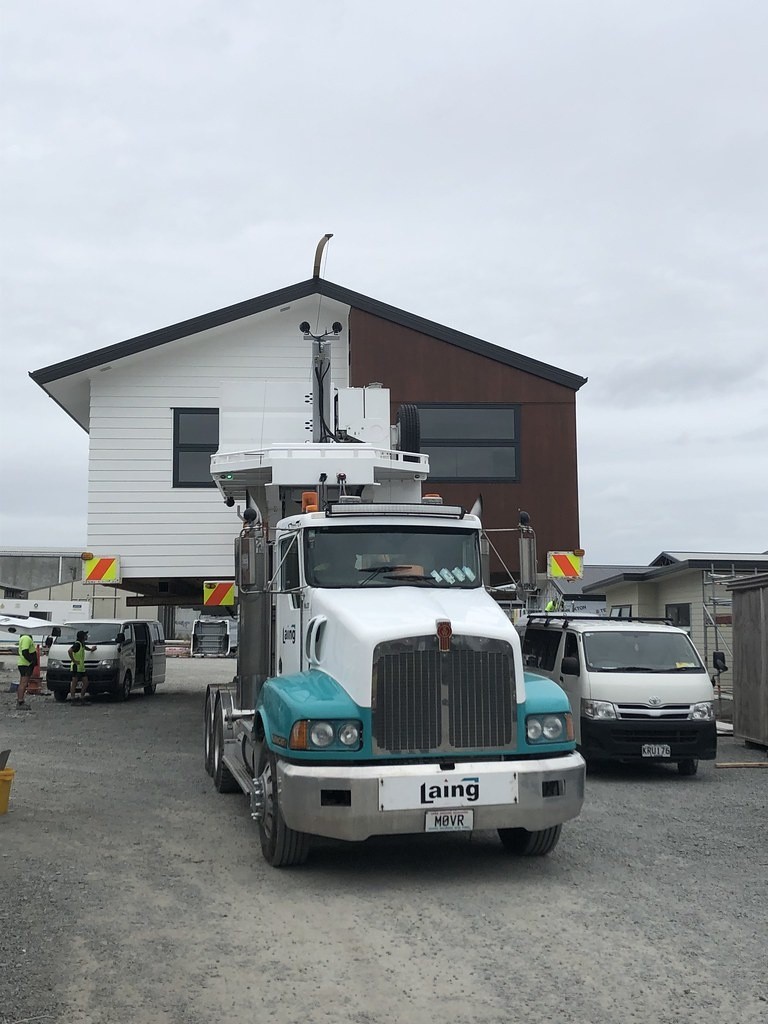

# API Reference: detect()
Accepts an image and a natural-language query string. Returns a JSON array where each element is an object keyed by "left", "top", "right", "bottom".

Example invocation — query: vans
[
  {"left": 45, "top": 620, "right": 170, "bottom": 701},
  {"left": 517, "top": 612, "right": 729, "bottom": 775}
]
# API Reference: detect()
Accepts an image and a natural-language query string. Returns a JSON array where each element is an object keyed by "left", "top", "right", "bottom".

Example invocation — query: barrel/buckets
[{"left": 0, "top": 768, "right": 15, "bottom": 816}]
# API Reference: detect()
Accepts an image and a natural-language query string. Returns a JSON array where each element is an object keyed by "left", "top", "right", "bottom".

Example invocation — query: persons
[
  {"left": 16, "top": 634, "right": 38, "bottom": 711},
  {"left": 68, "top": 631, "right": 98, "bottom": 706}
]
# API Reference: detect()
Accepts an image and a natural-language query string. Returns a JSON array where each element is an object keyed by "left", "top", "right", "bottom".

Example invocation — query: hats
[{"left": 76, "top": 631, "right": 88, "bottom": 640}]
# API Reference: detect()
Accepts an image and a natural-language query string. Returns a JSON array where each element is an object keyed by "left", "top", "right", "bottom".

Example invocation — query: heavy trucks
[
  {"left": 0, "top": 598, "right": 91, "bottom": 656},
  {"left": 202, "top": 320, "right": 587, "bottom": 870}
]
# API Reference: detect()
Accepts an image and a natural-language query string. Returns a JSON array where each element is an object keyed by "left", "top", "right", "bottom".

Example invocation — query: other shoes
[
  {"left": 70, "top": 699, "right": 82, "bottom": 706},
  {"left": 80, "top": 698, "right": 92, "bottom": 706},
  {"left": 15, "top": 699, "right": 31, "bottom": 711}
]
